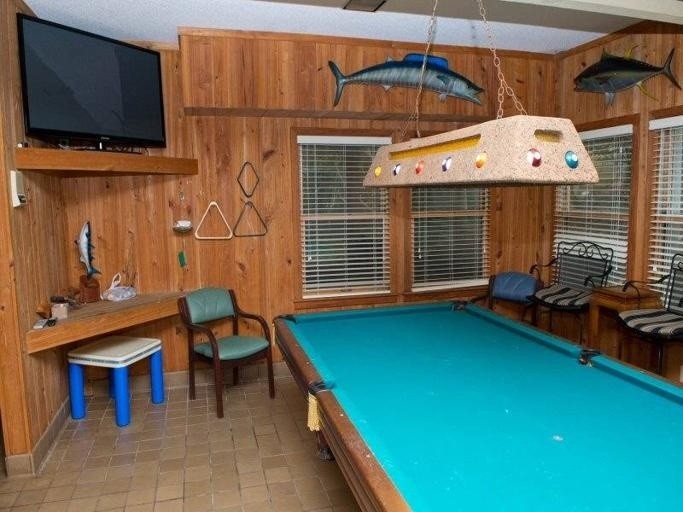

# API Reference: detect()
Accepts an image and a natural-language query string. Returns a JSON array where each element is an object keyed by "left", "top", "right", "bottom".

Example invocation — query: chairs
[
  {"left": 178, "top": 287, "right": 275, "bottom": 418},
  {"left": 619, "top": 253, "right": 683, "bottom": 376},
  {"left": 526, "top": 240, "right": 614, "bottom": 345}
]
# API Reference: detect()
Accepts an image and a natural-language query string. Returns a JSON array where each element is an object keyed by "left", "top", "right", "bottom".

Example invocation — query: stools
[{"left": 488, "top": 271, "right": 540, "bottom": 326}]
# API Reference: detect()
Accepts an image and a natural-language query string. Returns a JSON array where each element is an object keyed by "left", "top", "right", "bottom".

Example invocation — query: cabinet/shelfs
[{"left": 13, "top": 149, "right": 199, "bottom": 355}]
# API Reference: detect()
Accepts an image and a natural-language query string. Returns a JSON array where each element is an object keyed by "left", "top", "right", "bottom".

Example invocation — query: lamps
[{"left": 359, "top": 0, "right": 600, "bottom": 188}]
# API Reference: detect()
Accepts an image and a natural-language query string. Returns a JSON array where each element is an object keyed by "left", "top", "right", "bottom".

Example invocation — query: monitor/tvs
[{"left": 17, "top": 13, "right": 166, "bottom": 154}]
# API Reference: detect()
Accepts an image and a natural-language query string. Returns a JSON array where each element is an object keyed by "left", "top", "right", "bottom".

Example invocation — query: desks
[
  {"left": 67, "top": 335, "right": 163, "bottom": 427},
  {"left": 272, "top": 298, "right": 683, "bottom": 511}
]
